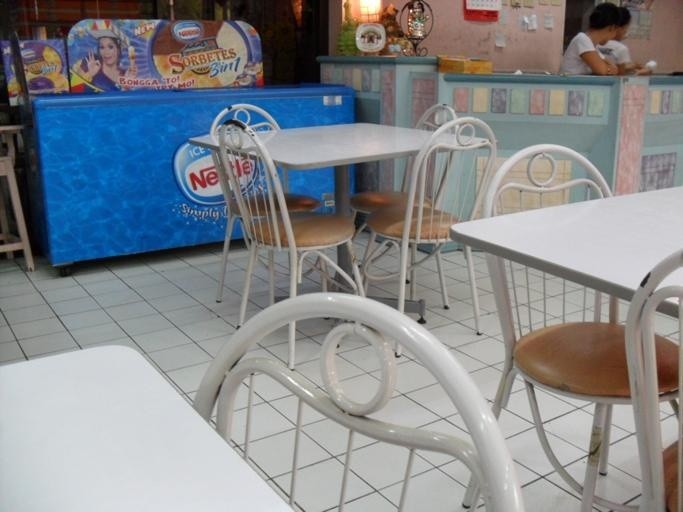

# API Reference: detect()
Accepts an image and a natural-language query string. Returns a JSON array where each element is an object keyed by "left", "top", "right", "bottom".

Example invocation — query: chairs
[
  {"left": 190, "top": 289, "right": 527, "bottom": 512},
  {"left": 358, "top": 116, "right": 496, "bottom": 358},
  {"left": 624, "top": 247, "right": 682, "bottom": 511},
  {"left": 345, "top": 101, "right": 458, "bottom": 308},
  {"left": 218, "top": 118, "right": 369, "bottom": 371},
  {"left": 461, "top": 142, "right": 680, "bottom": 511},
  {"left": 209, "top": 101, "right": 320, "bottom": 304}
]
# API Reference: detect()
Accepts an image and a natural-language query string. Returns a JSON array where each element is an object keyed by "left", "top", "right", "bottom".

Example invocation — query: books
[{"left": 465, "top": 0, "right": 501, "bottom": 11}]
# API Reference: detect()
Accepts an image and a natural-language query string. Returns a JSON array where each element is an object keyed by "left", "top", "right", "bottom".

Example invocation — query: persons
[
  {"left": 79, "top": 29, "right": 138, "bottom": 92},
  {"left": 558, "top": 2, "right": 653, "bottom": 76}
]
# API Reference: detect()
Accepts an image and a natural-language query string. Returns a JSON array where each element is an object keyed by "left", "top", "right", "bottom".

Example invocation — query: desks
[
  {"left": 188, "top": 123, "right": 489, "bottom": 323},
  {"left": 450, "top": 184, "right": 683, "bottom": 326},
  {"left": 0, "top": 343, "right": 296, "bottom": 511}
]
